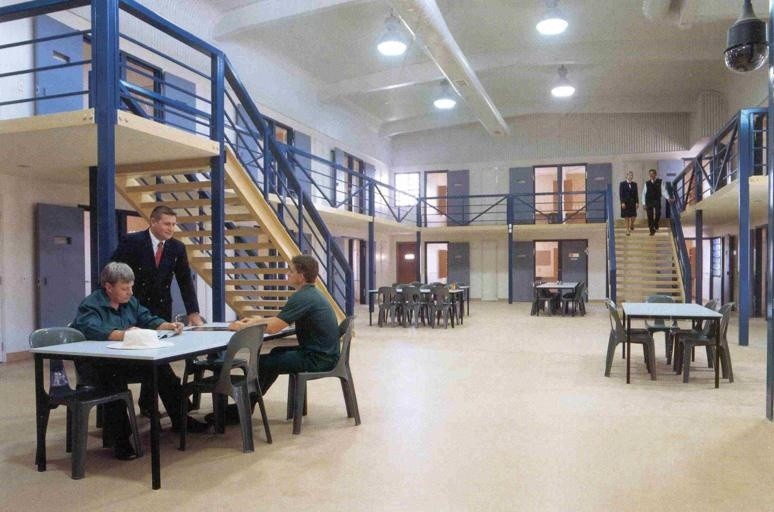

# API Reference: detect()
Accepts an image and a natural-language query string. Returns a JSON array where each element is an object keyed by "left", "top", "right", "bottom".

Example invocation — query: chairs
[
  {"left": 180, "top": 324, "right": 273, "bottom": 453},
  {"left": 530, "top": 279, "right": 588, "bottom": 316},
  {"left": 666, "top": 299, "right": 720, "bottom": 366},
  {"left": 605, "top": 297, "right": 657, "bottom": 381},
  {"left": 644, "top": 295, "right": 680, "bottom": 358},
  {"left": 28, "top": 326, "right": 142, "bottom": 479},
  {"left": 175, "top": 314, "right": 248, "bottom": 434},
  {"left": 286, "top": 315, "right": 360, "bottom": 434},
  {"left": 674, "top": 303, "right": 736, "bottom": 383},
  {"left": 368, "top": 281, "right": 470, "bottom": 329}
]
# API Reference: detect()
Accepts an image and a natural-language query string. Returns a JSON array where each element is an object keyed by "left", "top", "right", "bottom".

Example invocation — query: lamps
[
  {"left": 433, "top": 78, "right": 457, "bottom": 110},
  {"left": 550, "top": 65, "right": 576, "bottom": 98},
  {"left": 377, "top": 8, "right": 407, "bottom": 57},
  {"left": 533, "top": 0, "right": 568, "bottom": 36}
]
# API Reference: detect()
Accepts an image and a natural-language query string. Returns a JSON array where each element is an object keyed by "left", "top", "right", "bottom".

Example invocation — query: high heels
[
  {"left": 631, "top": 226, "right": 633, "bottom": 230},
  {"left": 626, "top": 230, "right": 630, "bottom": 236}
]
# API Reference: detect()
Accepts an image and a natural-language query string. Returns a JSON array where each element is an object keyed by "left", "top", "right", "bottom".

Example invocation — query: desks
[
  {"left": 32, "top": 323, "right": 294, "bottom": 491},
  {"left": 622, "top": 302, "right": 723, "bottom": 388}
]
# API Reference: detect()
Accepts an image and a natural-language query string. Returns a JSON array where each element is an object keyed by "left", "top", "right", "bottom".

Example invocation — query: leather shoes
[
  {"left": 109, "top": 439, "right": 137, "bottom": 460},
  {"left": 649, "top": 230, "right": 656, "bottom": 235},
  {"left": 204, "top": 405, "right": 240, "bottom": 425},
  {"left": 170, "top": 417, "right": 212, "bottom": 431},
  {"left": 137, "top": 397, "right": 163, "bottom": 418},
  {"left": 655, "top": 223, "right": 659, "bottom": 230}
]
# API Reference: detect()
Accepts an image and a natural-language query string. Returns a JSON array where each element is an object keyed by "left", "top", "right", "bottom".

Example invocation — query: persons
[
  {"left": 640, "top": 168, "right": 674, "bottom": 235},
  {"left": 203, "top": 253, "right": 340, "bottom": 426},
  {"left": 98, "top": 204, "right": 210, "bottom": 433},
  {"left": 70, "top": 261, "right": 213, "bottom": 460},
  {"left": 619, "top": 171, "right": 640, "bottom": 236}
]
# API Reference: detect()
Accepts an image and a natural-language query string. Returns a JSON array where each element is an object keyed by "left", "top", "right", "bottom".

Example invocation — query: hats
[{"left": 106, "top": 329, "right": 173, "bottom": 349}]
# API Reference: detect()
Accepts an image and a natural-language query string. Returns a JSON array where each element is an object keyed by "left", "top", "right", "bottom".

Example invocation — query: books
[{"left": 156, "top": 328, "right": 179, "bottom": 341}]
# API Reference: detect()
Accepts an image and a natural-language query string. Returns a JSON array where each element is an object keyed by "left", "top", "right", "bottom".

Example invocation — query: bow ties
[{"left": 627, "top": 183, "right": 632, "bottom": 185}]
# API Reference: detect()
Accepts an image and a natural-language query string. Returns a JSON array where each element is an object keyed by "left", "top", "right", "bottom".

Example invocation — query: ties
[
  {"left": 155, "top": 242, "right": 163, "bottom": 270},
  {"left": 653, "top": 179, "right": 655, "bottom": 184}
]
